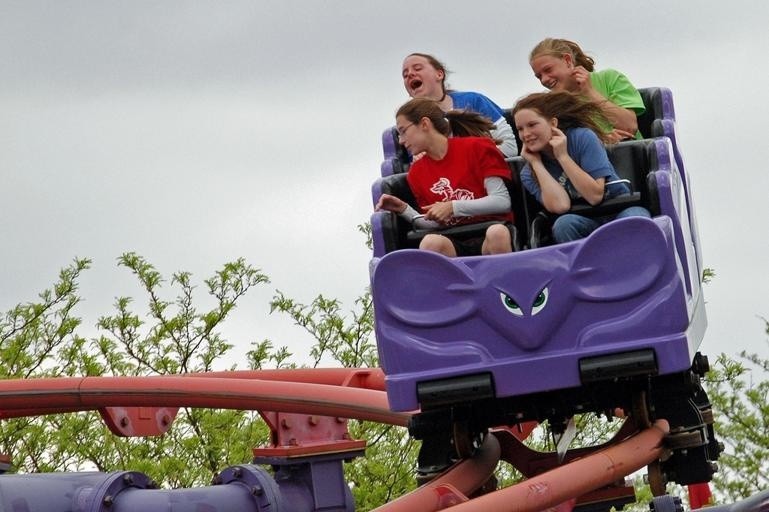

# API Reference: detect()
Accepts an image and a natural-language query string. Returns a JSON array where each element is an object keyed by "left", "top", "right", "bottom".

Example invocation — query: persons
[
  {"left": 371, "top": 96, "right": 517, "bottom": 258},
  {"left": 511, "top": 92, "right": 653, "bottom": 245},
  {"left": 529, "top": 38, "right": 648, "bottom": 145},
  {"left": 401, "top": 54, "right": 520, "bottom": 165}
]
